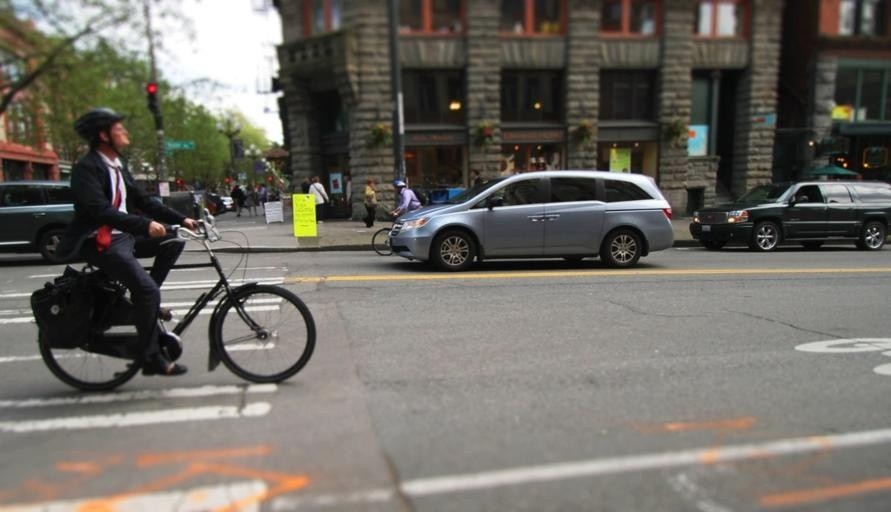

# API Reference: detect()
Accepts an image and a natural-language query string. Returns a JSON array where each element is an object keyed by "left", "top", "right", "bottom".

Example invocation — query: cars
[{"left": 193, "top": 189, "right": 233, "bottom": 215}]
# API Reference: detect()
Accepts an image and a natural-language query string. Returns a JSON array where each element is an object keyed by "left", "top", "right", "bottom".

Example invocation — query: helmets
[
  {"left": 73, "top": 108, "right": 127, "bottom": 137},
  {"left": 392, "top": 180, "right": 405, "bottom": 187}
]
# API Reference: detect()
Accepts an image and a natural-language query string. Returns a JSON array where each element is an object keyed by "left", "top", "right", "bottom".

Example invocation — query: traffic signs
[{"left": 165, "top": 140, "right": 195, "bottom": 149}]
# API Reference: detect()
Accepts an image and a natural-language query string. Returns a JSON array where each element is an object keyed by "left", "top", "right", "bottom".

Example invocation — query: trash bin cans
[{"left": 687, "top": 186, "right": 704, "bottom": 217}]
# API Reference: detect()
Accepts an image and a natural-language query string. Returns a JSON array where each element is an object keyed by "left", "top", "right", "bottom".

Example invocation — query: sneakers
[
  {"left": 157, "top": 306, "right": 172, "bottom": 321},
  {"left": 143, "top": 363, "right": 188, "bottom": 375}
]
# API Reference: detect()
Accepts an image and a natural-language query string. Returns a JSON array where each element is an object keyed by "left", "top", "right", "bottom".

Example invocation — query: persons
[
  {"left": 362, "top": 179, "right": 378, "bottom": 227},
  {"left": 343, "top": 173, "right": 352, "bottom": 220},
  {"left": 302, "top": 177, "right": 310, "bottom": 193},
  {"left": 387, "top": 180, "right": 421, "bottom": 217},
  {"left": 230, "top": 183, "right": 279, "bottom": 217},
  {"left": 56, "top": 107, "right": 198, "bottom": 376},
  {"left": 308, "top": 177, "right": 330, "bottom": 224}
]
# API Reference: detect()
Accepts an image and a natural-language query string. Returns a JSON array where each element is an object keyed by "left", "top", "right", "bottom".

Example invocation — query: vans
[{"left": 389, "top": 167, "right": 674, "bottom": 270}]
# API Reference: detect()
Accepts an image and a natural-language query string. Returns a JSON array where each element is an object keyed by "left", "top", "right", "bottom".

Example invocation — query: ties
[{"left": 96, "top": 168, "right": 121, "bottom": 256}]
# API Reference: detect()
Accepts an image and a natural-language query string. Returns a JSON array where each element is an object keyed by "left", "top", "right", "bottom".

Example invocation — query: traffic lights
[{"left": 146, "top": 83, "right": 160, "bottom": 111}]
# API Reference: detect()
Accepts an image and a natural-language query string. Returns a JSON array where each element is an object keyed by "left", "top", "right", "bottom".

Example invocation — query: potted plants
[{"left": 476, "top": 119, "right": 496, "bottom": 147}]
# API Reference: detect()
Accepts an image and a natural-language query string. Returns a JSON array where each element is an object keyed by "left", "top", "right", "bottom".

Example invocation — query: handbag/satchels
[{"left": 31, "top": 278, "right": 93, "bottom": 344}]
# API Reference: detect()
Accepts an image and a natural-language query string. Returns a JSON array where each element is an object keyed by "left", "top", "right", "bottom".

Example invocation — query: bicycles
[
  {"left": 372, "top": 211, "right": 399, "bottom": 257},
  {"left": 37, "top": 206, "right": 315, "bottom": 392}
]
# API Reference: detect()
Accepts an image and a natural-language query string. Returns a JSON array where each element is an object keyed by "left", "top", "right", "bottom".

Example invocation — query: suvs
[
  {"left": 690, "top": 182, "right": 890, "bottom": 249},
  {"left": 0, "top": 180, "right": 77, "bottom": 262}
]
